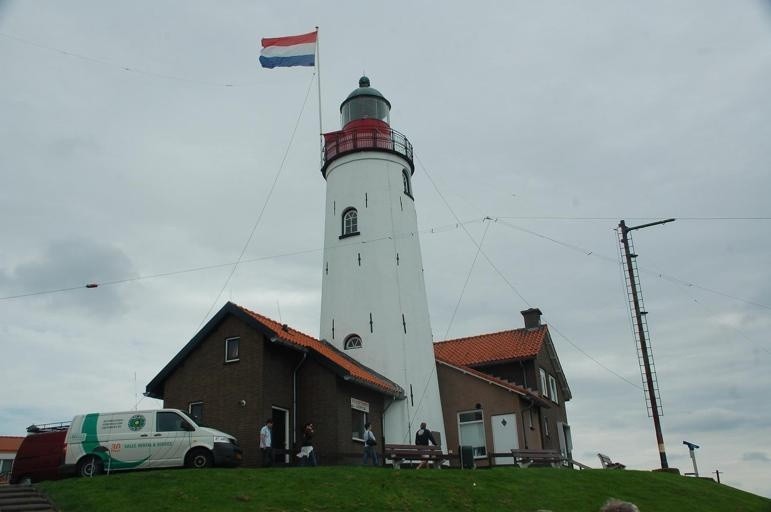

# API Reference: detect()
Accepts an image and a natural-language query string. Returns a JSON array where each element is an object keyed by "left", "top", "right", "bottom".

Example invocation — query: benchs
[
  {"left": 384, "top": 444, "right": 452, "bottom": 470},
  {"left": 597, "top": 453, "right": 627, "bottom": 472},
  {"left": 510, "top": 449, "right": 567, "bottom": 468}
]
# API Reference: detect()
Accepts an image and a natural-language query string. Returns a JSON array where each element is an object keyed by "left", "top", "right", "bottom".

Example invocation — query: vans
[
  {"left": 8, "top": 421, "right": 72, "bottom": 485},
  {"left": 61, "top": 408, "right": 242, "bottom": 477}
]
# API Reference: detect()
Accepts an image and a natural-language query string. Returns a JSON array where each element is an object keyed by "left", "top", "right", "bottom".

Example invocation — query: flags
[{"left": 259, "top": 31, "right": 318, "bottom": 70}]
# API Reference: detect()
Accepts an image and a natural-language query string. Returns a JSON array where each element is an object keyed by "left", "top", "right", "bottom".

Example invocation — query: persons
[
  {"left": 298, "top": 421, "right": 319, "bottom": 466},
  {"left": 599, "top": 497, "right": 641, "bottom": 512},
  {"left": 362, "top": 422, "right": 383, "bottom": 467},
  {"left": 414, "top": 423, "right": 438, "bottom": 469},
  {"left": 259, "top": 419, "right": 275, "bottom": 468}
]
